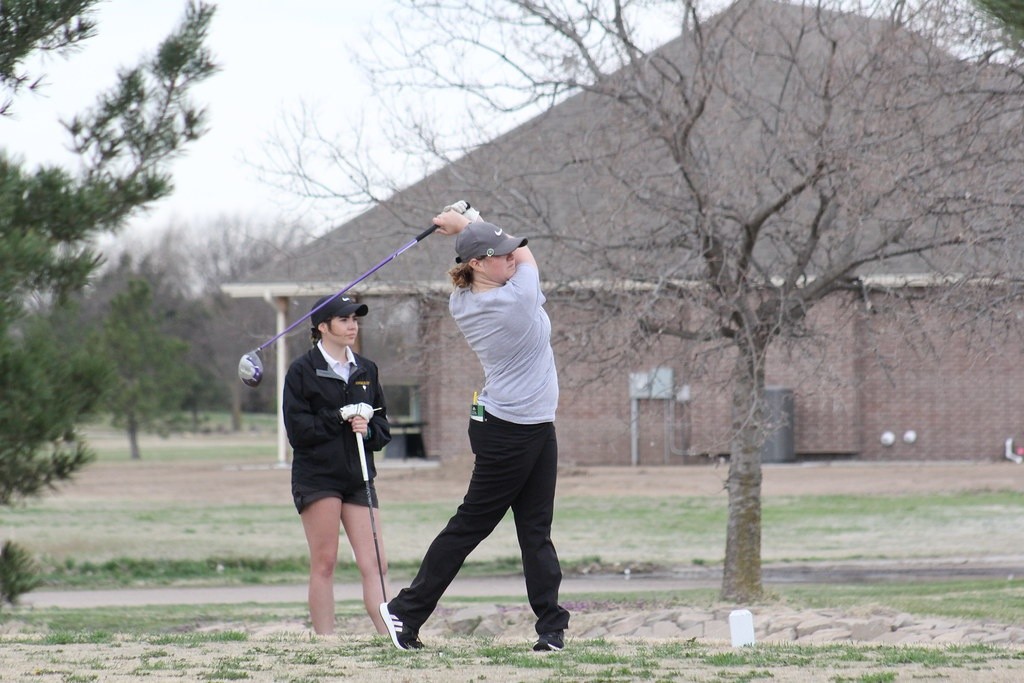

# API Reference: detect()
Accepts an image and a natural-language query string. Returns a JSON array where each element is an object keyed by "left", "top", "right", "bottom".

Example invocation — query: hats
[
  {"left": 311, "top": 293, "right": 368, "bottom": 327},
  {"left": 456, "top": 222, "right": 528, "bottom": 266}
]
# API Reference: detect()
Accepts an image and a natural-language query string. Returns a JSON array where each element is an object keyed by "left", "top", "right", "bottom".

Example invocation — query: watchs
[{"left": 364, "top": 424, "right": 372, "bottom": 439}]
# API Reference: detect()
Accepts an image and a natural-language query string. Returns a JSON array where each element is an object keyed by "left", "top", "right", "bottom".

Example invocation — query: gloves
[
  {"left": 443, "top": 200, "right": 480, "bottom": 225},
  {"left": 340, "top": 403, "right": 374, "bottom": 422}
]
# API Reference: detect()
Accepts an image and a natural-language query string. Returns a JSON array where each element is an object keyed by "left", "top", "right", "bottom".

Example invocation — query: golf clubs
[
  {"left": 236, "top": 200, "right": 473, "bottom": 387},
  {"left": 350, "top": 414, "right": 389, "bottom": 604}
]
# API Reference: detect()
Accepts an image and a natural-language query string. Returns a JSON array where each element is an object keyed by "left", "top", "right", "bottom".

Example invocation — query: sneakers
[
  {"left": 532, "top": 631, "right": 565, "bottom": 651},
  {"left": 379, "top": 602, "right": 427, "bottom": 652}
]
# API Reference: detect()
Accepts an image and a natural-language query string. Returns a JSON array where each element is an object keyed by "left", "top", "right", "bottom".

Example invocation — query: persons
[
  {"left": 281, "top": 292, "right": 394, "bottom": 637},
  {"left": 378, "top": 200, "right": 572, "bottom": 654}
]
